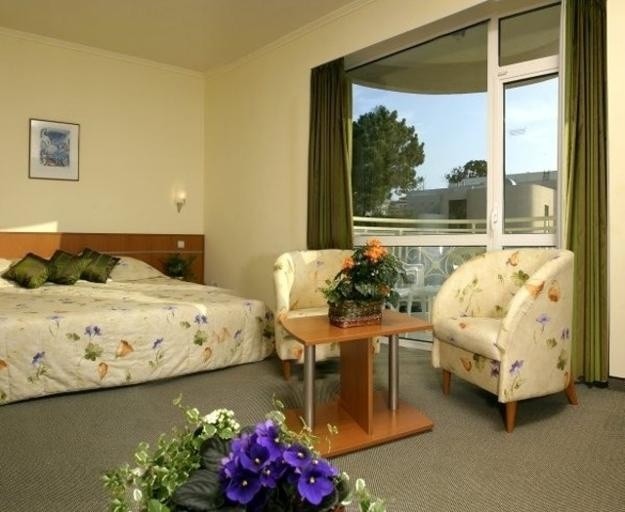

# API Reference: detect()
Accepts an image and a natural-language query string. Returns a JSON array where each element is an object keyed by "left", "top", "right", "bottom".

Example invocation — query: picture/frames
[{"left": 28, "top": 118, "right": 81, "bottom": 181}]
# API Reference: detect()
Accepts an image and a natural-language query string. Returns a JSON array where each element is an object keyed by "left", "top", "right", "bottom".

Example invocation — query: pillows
[
  {"left": 48, "top": 249, "right": 86, "bottom": 285},
  {"left": 80, "top": 247, "right": 120, "bottom": 282},
  {"left": 1, "top": 250, "right": 50, "bottom": 289}
]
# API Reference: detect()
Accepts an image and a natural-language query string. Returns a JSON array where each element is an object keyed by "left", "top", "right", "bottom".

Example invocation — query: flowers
[
  {"left": 320, "top": 239, "right": 411, "bottom": 310},
  {"left": 101, "top": 393, "right": 390, "bottom": 511}
]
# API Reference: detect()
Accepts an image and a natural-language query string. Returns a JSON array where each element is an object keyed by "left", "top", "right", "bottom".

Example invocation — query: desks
[{"left": 278, "top": 308, "right": 435, "bottom": 458}]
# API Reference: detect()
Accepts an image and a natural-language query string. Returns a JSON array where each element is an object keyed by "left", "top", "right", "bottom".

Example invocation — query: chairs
[
  {"left": 430, "top": 247, "right": 580, "bottom": 433},
  {"left": 391, "top": 258, "right": 427, "bottom": 313},
  {"left": 273, "top": 249, "right": 380, "bottom": 381}
]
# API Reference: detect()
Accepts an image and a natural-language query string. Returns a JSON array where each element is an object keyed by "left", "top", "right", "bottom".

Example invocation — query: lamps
[{"left": 174, "top": 184, "right": 188, "bottom": 205}]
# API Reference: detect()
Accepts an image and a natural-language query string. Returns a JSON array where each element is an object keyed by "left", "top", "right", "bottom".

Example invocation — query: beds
[{"left": 0, "top": 255, "right": 274, "bottom": 405}]
